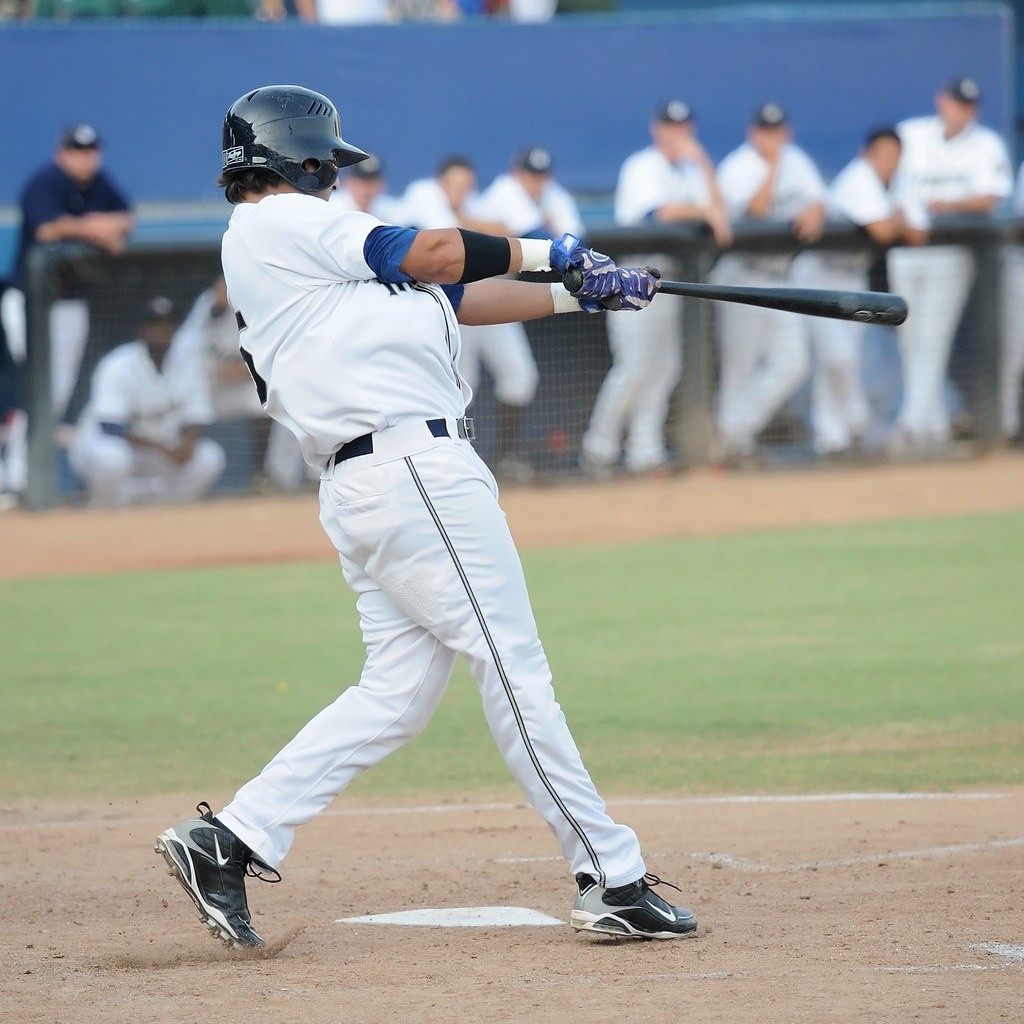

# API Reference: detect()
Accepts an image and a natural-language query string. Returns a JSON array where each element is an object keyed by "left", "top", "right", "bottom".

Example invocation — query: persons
[
  {"left": 152, "top": 83, "right": 698, "bottom": 959},
  {"left": 0, "top": 77, "right": 1024, "bottom": 512}
]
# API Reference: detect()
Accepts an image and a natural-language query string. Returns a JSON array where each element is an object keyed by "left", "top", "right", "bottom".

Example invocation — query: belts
[{"left": 329, "top": 418, "right": 477, "bottom": 466}]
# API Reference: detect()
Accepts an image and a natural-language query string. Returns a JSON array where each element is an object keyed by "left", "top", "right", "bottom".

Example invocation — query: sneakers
[
  {"left": 155, "top": 813, "right": 265, "bottom": 954},
  {"left": 570, "top": 872, "right": 699, "bottom": 939}
]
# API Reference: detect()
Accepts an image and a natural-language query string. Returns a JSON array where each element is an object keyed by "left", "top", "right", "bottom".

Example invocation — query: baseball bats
[{"left": 561, "top": 268, "right": 909, "bottom": 329}]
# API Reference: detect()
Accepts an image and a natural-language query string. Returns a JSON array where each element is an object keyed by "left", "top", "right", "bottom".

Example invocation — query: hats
[
  {"left": 518, "top": 146, "right": 555, "bottom": 175},
  {"left": 654, "top": 97, "right": 693, "bottom": 123},
  {"left": 948, "top": 76, "right": 982, "bottom": 103},
  {"left": 61, "top": 121, "right": 102, "bottom": 153},
  {"left": 752, "top": 104, "right": 788, "bottom": 128},
  {"left": 141, "top": 297, "right": 182, "bottom": 324},
  {"left": 351, "top": 153, "right": 386, "bottom": 181}
]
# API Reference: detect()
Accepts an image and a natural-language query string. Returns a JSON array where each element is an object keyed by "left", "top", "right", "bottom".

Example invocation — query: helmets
[{"left": 223, "top": 83, "right": 371, "bottom": 193}]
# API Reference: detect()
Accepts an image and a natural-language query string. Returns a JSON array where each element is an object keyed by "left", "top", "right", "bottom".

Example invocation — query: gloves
[{"left": 550, "top": 235, "right": 663, "bottom": 315}]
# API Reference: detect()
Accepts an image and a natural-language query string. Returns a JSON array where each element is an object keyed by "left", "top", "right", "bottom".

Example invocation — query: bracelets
[{"left": 454, "top": 228, "right": 511, "bottom": 286}]
